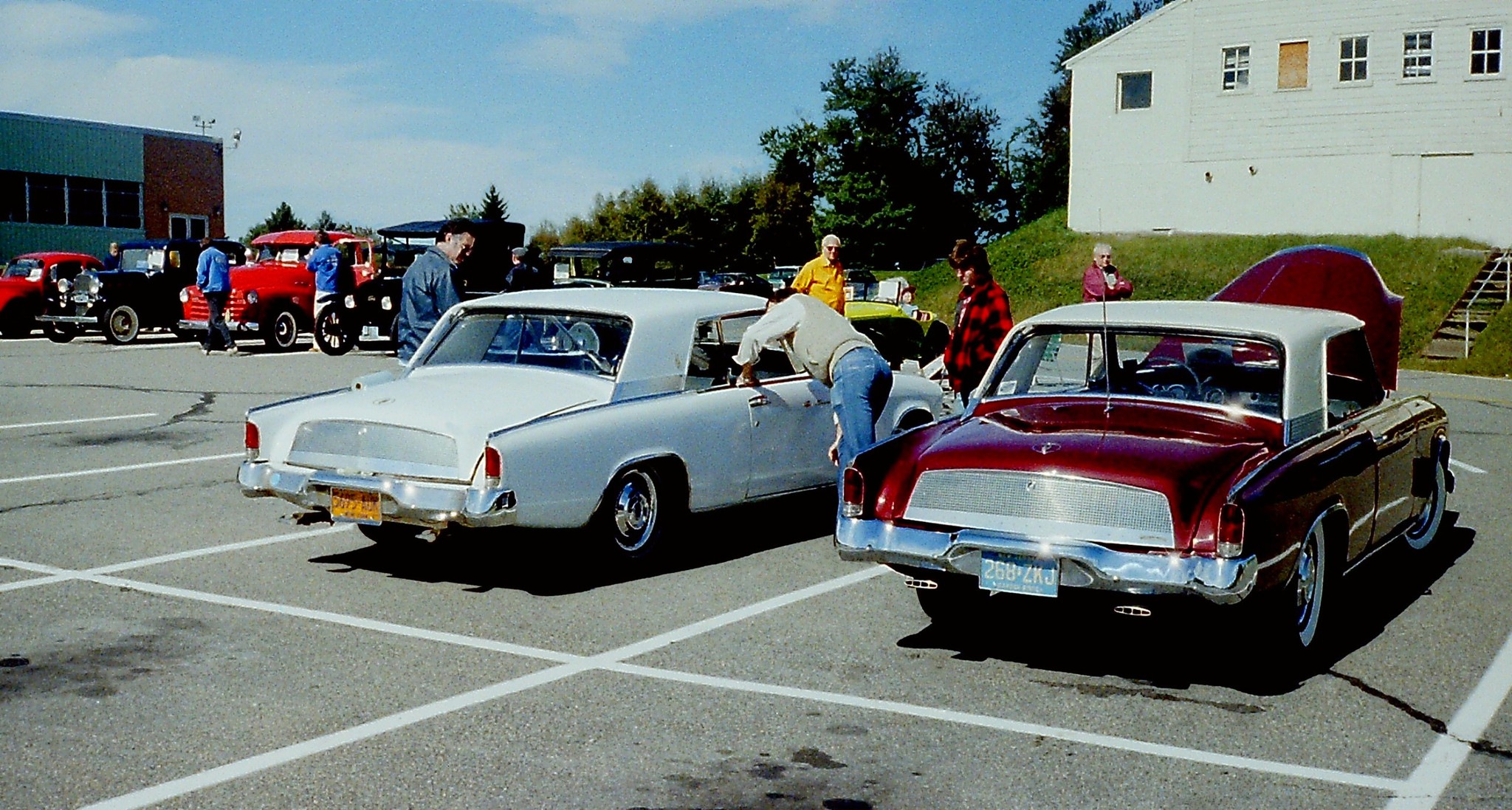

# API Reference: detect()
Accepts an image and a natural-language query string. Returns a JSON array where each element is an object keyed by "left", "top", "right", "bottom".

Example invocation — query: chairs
[{"left": 1187, "top": 347, "right": 1238, "bottom": 388}]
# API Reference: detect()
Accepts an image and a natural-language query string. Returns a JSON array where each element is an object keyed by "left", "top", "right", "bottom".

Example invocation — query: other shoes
[
  {"left": 309, "top": 347, "right": 322, "bottom": 352},
  {"left": 199, "top": 347, "right": 209, "bottom": 355},
  {"left": 224, "top": 345, "right": 238, "bottom": 355}
]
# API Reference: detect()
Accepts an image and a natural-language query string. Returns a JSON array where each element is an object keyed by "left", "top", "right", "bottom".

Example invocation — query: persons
[
  {"left": 732, "top": 287, "right": 893, "bottom": 466},
  {"left": 196, "top": 237, "right": 238, "bottom": 357},
  {"left": 1082, "top": 243, "right": 1133, "bottom": 375},
  {"left": 102, "top": 243, "right": 126, "bottom": 271},
  {"left": 506, "top": 247, "right": 540, "bottom": 292},
  {"left": 942, "top": 239, "right": 1014, "bottom": 409},
  {"left": 398, "top": 217, "right": 478, "bottom": 367},
  {"left": 790, "top": 234, "right": 845, "bottom": 316},
  {"left": 306, "top": 229, "right": 342, "bottom": 353}
]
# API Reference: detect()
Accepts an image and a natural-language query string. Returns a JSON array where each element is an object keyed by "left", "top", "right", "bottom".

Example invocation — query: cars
[
  {"left": 235, "top": 289, "right": 945, "bottom": 574},
  {"left": 826, "top": 300, "right": 957, "bottom": 385},
  {"left": 768, "top": 265, "right": 807, "bottom": 290},
  {"left": 843, "top": 268, "right": 879, "bottom": 290},
  {"left": 700, "top": 269, "right": 781, "bottom": 294},
  {"left": 0, "top": 251, "right": 105, "bottom": 338},
  {"left": 815, "top": 238, "right": 1461, "bottom": 667}
]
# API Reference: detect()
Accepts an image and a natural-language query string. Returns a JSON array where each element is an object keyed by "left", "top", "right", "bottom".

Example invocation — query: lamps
[
  {"left": 161, "top": 200, "right": 169, "bottom": 213},
  {"left": 214, "top": 203, "right": 222, "bottom": 215}
]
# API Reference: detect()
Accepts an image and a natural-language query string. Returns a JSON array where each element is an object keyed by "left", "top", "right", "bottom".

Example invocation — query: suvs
[
  {"left": 314, "top": 220, "right": 537, "bottom": 356},
  {"left": 35, "top": 236, "right": 247, "bottom": 346},
  {"left": 176, "top": 230, "right": 376, "bottom": 352},
  {"left": 542, "top": 240, "right": 710, "bottom": 289}
]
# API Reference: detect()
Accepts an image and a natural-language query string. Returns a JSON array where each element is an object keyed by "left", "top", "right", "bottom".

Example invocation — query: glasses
[
  {"left": 826, "top": 246, "right": 841, "bottom": 252},
  {"left": 1098, "top": 254, "right": 1111, "bottom": 259}
]
